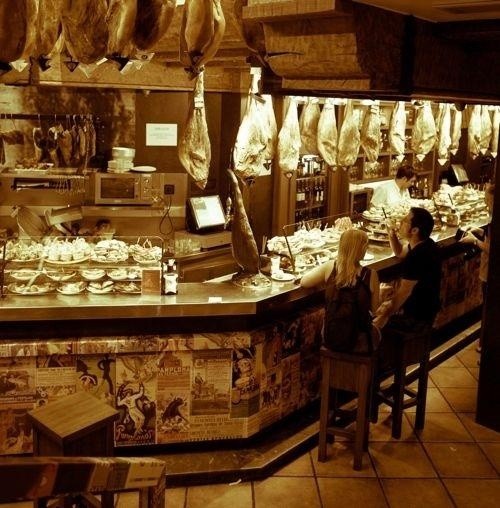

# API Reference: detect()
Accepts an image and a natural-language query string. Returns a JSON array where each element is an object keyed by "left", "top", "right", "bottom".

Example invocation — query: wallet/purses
[{"left": 455, "top": 229, "right": 480, "bottom": 246}]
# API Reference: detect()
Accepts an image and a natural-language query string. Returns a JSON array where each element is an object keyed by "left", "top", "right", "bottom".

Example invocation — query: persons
[
  {"left": 458, "top": 187, "right": 495, "bottom": 365},
  {"left": 369, "top": 166, "right": 417, "bottom": 214},
  {"left": 378, "top": 207, "right": 442, "bottom": 340},
  {"left": 302, "top": 229, "right": 383, "bottom": 355}
]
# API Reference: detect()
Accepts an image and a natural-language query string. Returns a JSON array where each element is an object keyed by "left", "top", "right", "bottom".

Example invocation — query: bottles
[{"left": 447, "top": 209, "right": 459, "bottom": 226}]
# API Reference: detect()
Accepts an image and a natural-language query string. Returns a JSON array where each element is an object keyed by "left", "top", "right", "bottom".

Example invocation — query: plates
[
  {"left": 271, "top": 272, "right": 294, "bottom": 281},
  {"left": 132, "top": 166, "right": 157, "bottom": 172}
]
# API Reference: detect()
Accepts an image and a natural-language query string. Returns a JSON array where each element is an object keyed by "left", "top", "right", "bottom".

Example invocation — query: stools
[
  {"left": 318, "top": 328, "right": 432, "bottom": 471},
  {"left": 26, "top": 391, "right": 120, "bottom": 508}
]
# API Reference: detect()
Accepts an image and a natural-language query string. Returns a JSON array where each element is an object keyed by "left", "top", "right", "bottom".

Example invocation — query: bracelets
[{"left": 474, "top": 239, "right": 478, "bottom": 245}]
